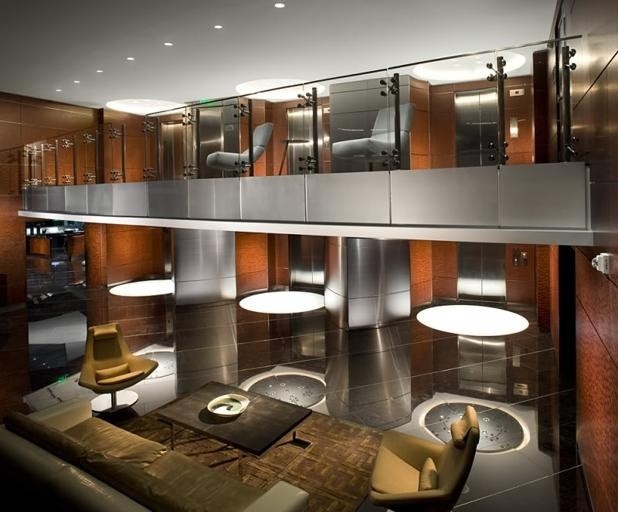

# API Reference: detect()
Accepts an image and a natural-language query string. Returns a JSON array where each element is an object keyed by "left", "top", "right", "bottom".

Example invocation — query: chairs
[
  {"left": 332, "top": 103, "right": 416, "bottom": 172},
  {"left": 367, "top": 403, "right": 479, "bottom": 512},
  {"left": 78, "top": 322, "right": 158, "bottom": 412},
  {"left": 206, "top": 122, "right": 274, "bottom": 178}
]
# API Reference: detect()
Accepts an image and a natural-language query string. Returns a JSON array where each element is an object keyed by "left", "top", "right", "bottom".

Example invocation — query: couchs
[{"left": 3, "top": 399, "right": 310, "bottom": 510}]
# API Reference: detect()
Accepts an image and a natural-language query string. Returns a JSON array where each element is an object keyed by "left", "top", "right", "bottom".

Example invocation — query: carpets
[{"left": 119, "top": 381, "right": 384, "bottom": 511}]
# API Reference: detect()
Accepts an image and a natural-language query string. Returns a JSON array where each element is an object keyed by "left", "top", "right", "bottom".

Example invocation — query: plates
[{"left": 207, "top": 393, "right": 250, "bottom": 418}]
